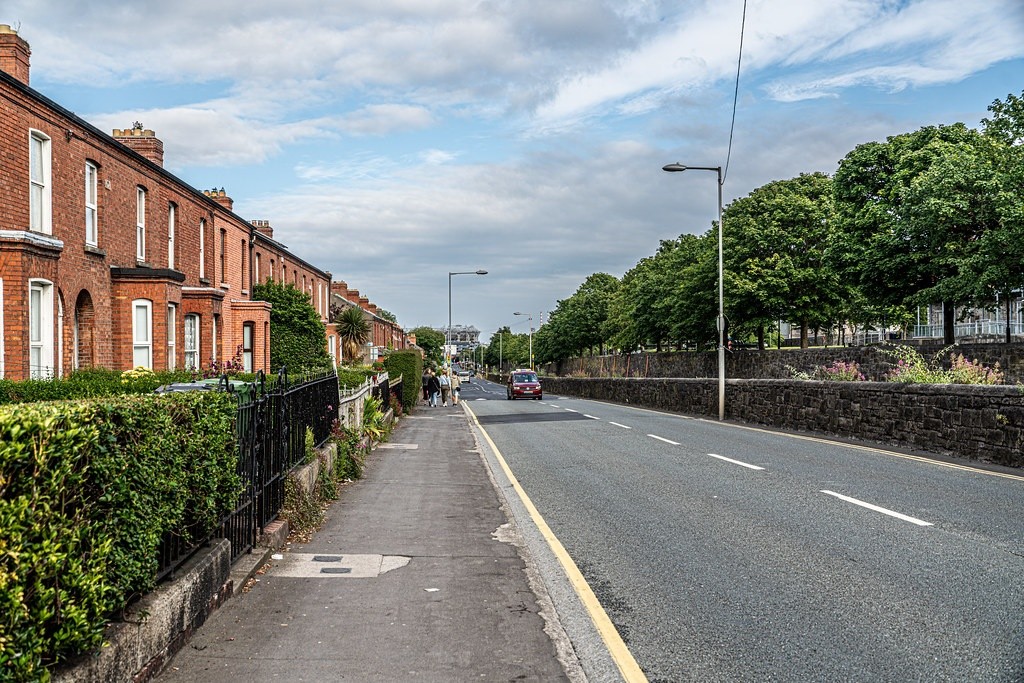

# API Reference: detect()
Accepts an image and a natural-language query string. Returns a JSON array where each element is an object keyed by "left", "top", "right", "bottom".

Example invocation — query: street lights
[
  {"left": 513, "top": 312, "right": 532, "bottom": 370},
  {"left": 490, "top": 332, "right": 503, "bottom": 385},
  {"left": 662, "top": 161, "right": 728, "bottom": 424},
  {"left": 447, "top": 269, "right": 489, "bottom": 328}
]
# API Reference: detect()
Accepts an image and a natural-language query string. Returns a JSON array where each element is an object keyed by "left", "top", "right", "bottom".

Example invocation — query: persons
[
  {"left": 421, "top": 368, "right": 433, "bottom": 406},
  {"left": 449, "top": 370, "right": 462, "bottom": 407},
  {"left": 427, "top": 371, "right": 441, "bottom": 407},
  {"left": 440, "top": 369, "right": 451, "bottom": 407}
]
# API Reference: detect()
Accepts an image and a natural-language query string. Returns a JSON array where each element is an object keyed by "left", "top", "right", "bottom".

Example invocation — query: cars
[{"left": 507, "top": 368, "right": 543, "bottom": 401}]
